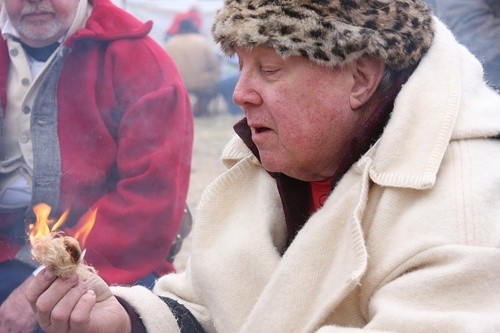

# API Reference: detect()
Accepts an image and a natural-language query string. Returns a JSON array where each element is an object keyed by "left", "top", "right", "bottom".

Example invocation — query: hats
[{"left": 209, "top": 0, "right": 434, "bottom": 70}]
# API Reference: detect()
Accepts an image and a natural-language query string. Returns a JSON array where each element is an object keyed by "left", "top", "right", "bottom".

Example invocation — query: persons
[
  {"left": 0, "top": 0, "right": 194, "bottom": 333},
  {"left": 25, "top": 0, "right": 500, "bottom": 331},
  {"left": 163, "top": 21, "right": 220, "bottom": 117}
]
